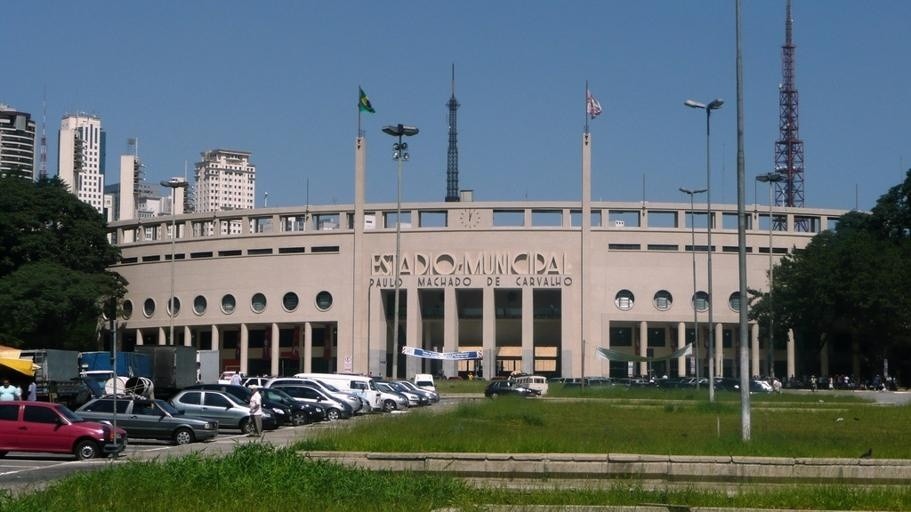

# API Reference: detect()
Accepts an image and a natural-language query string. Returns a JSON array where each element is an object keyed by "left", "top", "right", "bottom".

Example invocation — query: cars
[
  {"left": 76, "top": 395, "right": 220, "bottom": 445},
  {"left": 546, "top": 374, "right": 784, "bottom": 394},
  {"left": 485, "top": 379, "right": 541, "bottom": 399},
  {"left": 171, "top": 372, "right": 437, "bottom": 437}
]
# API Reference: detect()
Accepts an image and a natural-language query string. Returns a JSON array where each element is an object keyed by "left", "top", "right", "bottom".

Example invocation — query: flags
[
  {"left": 586, "top": 89, "right": 603, "bottom": 120},
  {"left": 358, "top": 87, "right": 376, "bottom": 114}
]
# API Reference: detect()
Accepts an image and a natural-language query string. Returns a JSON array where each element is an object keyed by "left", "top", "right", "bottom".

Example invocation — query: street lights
[
  {"left": 755, "top": 171, "right": 783, "bottom": 381},
  {"left": 102, "top": 293, "right": 127, "bottom": 458},
  {"left": 160, "top": 178, "right": 188, "bottom": 346},
  {"left": 685, "top": 95, "right": 723, "bottom": 400},
  {"left": 383, "top": 122, "right": 419, "bottom": 378},
  {"left": 680, "top": 186, "right": 707, "bottom": 391}
]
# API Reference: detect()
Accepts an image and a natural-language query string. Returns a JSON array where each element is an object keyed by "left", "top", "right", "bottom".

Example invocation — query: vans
[{"left": 514, "top": 376, "right": 547, "bottom": 394}]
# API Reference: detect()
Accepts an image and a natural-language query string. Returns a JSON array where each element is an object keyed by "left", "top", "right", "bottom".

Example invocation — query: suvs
[{"left": 0, "top": 399, "right": 128, "bottom": 461}]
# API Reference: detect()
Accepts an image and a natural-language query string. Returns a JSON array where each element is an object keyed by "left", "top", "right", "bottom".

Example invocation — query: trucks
[{"left": 0, "top": 343, "right": 221, "bottom": 402}]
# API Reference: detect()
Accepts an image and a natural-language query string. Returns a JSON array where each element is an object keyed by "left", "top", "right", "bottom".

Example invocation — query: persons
[
  {"left": 246, "top": 387, "right": 263, "bottom": 437},
  {"left": 0, "top": 376, "right": 37, "bottom": 401},
  {"left": 772, "top": 373, "right": 897, "bottom": 394},
  {"left": 230, "top": 371, "right": 242, "bottom": 386},
  {"left": 467, "top": 372, "right": 474, "bottom": 380}
]
